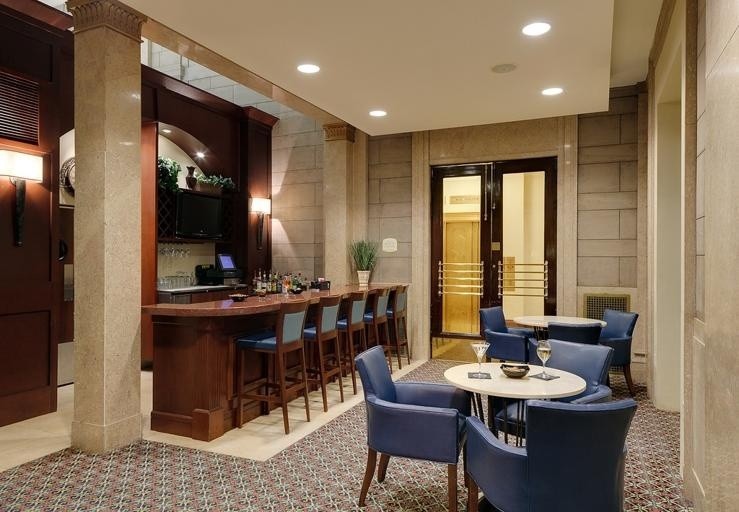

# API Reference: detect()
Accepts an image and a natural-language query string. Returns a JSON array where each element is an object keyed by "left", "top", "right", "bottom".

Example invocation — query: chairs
[
  {"left": 355, "top": 346, "right": 469, "bottom": 512},
  {"left": 477, "top": 308, "right": 545, "bottom": 365},
  {"left": 363, "top": 287, "right": 391, "bottom": 374},
  {"left": 598, "top": 309, "right": 638, "bottom": 398},
  {"left": 466, "top": 399, "right": 638, "bottom": 512},
  {"left": 493, "top": 340, "right": 614, "bottom": 443},
  {"left": 336, "top": 292, "right": 367, "bottom": 395},
  {"left": 303, "top": 295, "right": 342, "bottom": 414},
  {"left": 234, "top": 300, "right": 310, "bottom": 435},
  {"left": 386, "top": 284, "right": 411, "bottom": 369}
]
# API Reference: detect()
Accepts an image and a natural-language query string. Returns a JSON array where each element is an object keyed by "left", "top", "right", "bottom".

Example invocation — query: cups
[
  {"left": 470, "top": 342, "right": 490, "bottom": 377},
  {"left": 536, "top": 341, "right": 551, "bottom": 378}
]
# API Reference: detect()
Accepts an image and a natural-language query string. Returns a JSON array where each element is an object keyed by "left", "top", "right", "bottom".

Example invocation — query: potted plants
[
  {"left": 199, "top": 175, "right": 234, "bottom": 197},
  {"left": 158, "top": 155, "right": 185, "bottom": 192},
  {"left": 350, "top": 239, "right": 378, "bottom": 292}
]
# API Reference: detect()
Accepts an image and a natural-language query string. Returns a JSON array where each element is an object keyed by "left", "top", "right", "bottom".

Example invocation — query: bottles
[
  {"left": 271, "top": 273, "right": 278, "bottom": 292},
  {"left": 257, "top": 268, "right": 262, "bottom": 290},
  {"left": 278, "top": 271, "right": 307, "bottom": 293},
  {"left": 267, "top": 274, "right": 272, "bottom": 293},
  {"left": 252, "top": 270, "right": 257, "bottom": 288},
  {"left": 262, "top": 270, "right": 267, "bottom": 289}
]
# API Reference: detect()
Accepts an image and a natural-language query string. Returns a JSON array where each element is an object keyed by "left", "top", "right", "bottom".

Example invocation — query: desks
[{"left": 514, "top": 315, "right": 607, "bottom": 346}]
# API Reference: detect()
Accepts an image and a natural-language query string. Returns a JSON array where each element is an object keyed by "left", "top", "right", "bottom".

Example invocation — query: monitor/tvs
[{"left": 217, "top": 253, "right": 237, "bottom": 272}]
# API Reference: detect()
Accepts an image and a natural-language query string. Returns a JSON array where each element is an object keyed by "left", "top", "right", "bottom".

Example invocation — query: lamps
[
  {"left": 0, "top": 148, "right": 44, "bottom": 249},
  {"left": 248, "top": 197, "right": 270, "bottom": 248}
]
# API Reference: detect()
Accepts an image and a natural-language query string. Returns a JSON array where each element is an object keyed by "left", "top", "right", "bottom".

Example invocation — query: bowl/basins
[
  {"left": 290, "top": 289, "right": 302, "bottom": 294},
  {"left": 229, "top": 295, "right": 249, "bottom": 302}
]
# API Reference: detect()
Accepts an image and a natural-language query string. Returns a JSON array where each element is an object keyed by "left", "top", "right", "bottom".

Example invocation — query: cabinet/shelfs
[{"left": 154, "top": 284, "right": 253, "bottom": 304}]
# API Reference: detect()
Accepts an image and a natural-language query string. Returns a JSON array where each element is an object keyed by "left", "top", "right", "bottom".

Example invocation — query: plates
[{"left": 500, "top": 364, "right": 530, "bottom": 377}]
